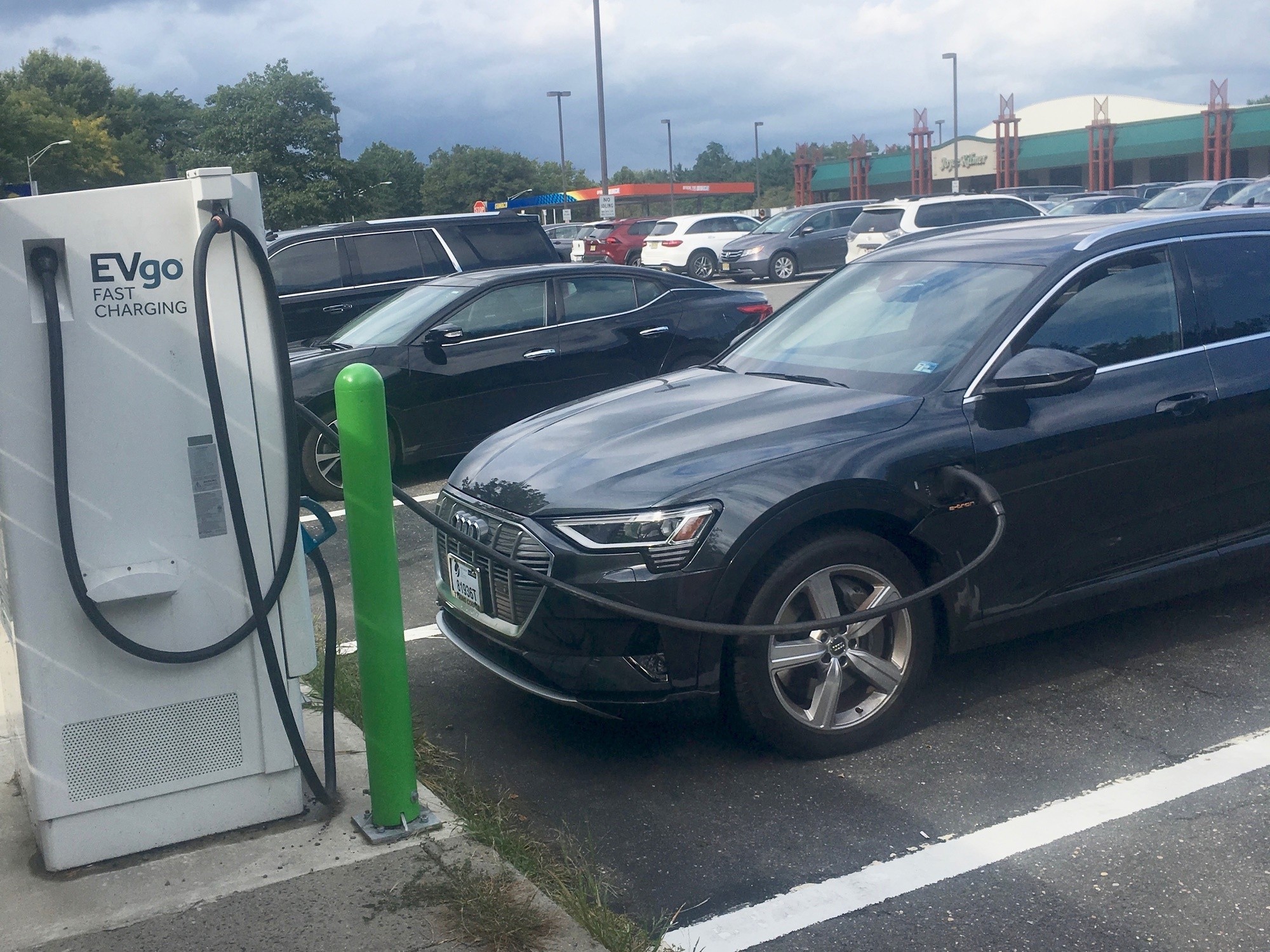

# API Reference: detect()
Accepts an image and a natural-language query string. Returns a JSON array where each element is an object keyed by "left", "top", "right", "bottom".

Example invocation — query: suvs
[
  {"left": 717, "top": 199, "right": 879, "bottom": 282},
  {"left": 583, "top": 217, "right": 669, "bottom": 269},
  {"left": 639, "top": 212, "right": 763, "bottom": 281},
  {"left": 263, "top": 211, "right": 563, "bottom": 339},
  {"left": 845, "top": 194, "right": 1053, "bottom": 263},
  {"left": 991, "top": 181, "right": 1188, "bottom": 214},
  {"left": 1124, "top": 177, "right": 1259, "bottom": 212}
]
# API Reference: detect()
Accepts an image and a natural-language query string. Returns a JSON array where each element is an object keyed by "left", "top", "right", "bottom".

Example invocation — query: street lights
[
  {"left": 935, "top": 119, "right": 945, "bottom": 145},
  {"left": 660, "top": 119, "right": 676, "bottom": 217},
  {"left": 546, "top": 90, "right": 572, "bottom": 224},
  {"left": 753, "top": 122, "right": 765, "bottom": 209},
  {"left": 942, "top": 52, "right": 962, "bottom": 194}
]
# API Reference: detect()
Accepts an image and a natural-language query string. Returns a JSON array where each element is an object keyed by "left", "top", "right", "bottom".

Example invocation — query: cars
[
  {"left": 1209, "top": 175, "right": 1270, "bottom": 212},
  {"left": 431, "top": 210, "right": 1270, "bottom": 758},
  {"left": 571, "top": 218, "right": 607, "bottom": 261},
  {"left": 538, "top": 222, "right": 584, "bottom": 261},
  {"left": 282, "top": 262, "right": 777, "bottom": 501}
]
